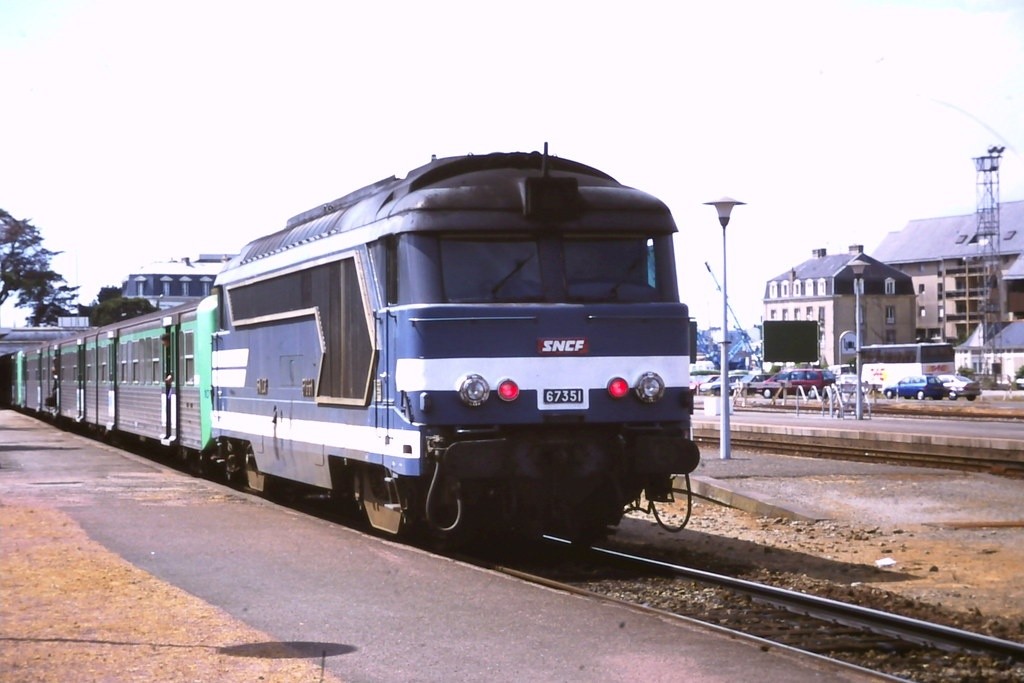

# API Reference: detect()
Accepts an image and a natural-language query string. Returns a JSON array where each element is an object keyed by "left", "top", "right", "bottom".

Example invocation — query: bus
[{"left": 860, "top": 343, "right": 955, "bottom": 391}]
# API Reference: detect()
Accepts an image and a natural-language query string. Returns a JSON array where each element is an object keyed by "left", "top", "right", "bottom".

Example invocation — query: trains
[{"left": 13, "top": 141, "right": 701, "bottom": 542}]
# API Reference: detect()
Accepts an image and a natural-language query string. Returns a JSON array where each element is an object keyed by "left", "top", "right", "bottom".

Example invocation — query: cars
[
  {"left": 936, "top": 375, "right": 982, "bottom": 400},
  {"left": 883, "top": 376, "right": 945, "bottom": 400},
  {"left": 691, "top": 374, "right": 773, "bottom": 396}
]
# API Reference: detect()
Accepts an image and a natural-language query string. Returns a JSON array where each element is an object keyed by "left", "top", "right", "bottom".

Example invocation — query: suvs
[{"left": 757, "top": 369, "right": 836, "bottom": 399}]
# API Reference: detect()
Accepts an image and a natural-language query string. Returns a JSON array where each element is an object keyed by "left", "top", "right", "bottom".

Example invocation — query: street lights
[
  {"left": 702, "top": 195, "right": 749, "bottom": 462},
  {"left": 846, "top": 257, "right": 871, "bottom": 421}
]
